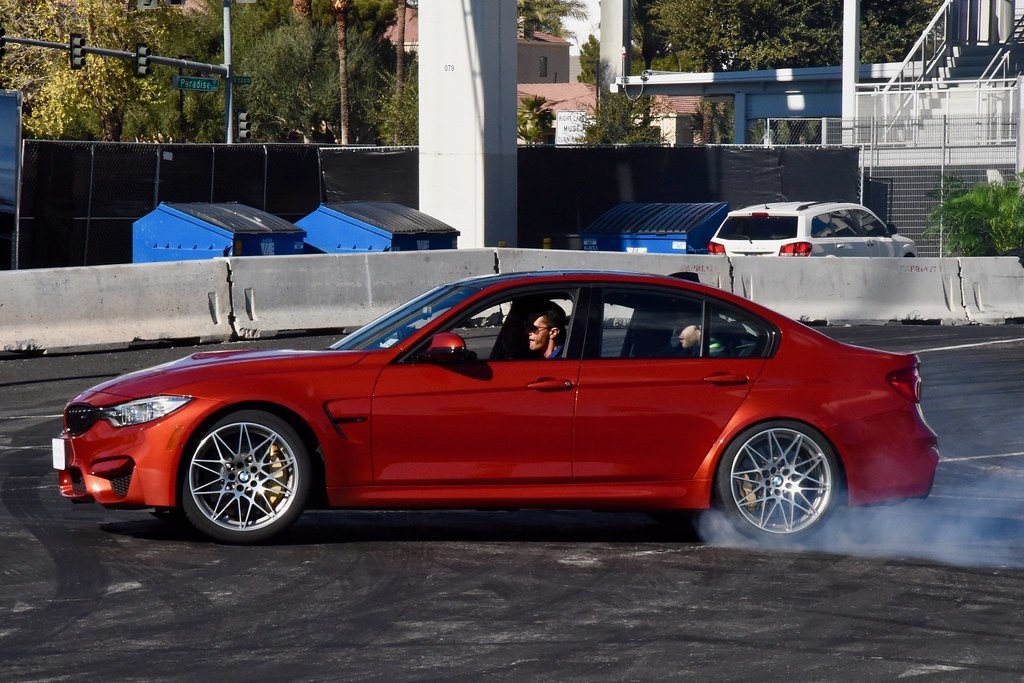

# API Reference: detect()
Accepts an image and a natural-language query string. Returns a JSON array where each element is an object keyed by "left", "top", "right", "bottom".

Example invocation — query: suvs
[{"left": 708, "top": 199, "right": 919, "bottom": 259}]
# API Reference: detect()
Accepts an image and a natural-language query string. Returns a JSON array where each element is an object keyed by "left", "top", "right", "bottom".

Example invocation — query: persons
[
  {"left": 525, "top": 299, "right": 568, "bottom": 361},
  {"left": 676, "top": 306, "right": 727, "bottom": 359}
]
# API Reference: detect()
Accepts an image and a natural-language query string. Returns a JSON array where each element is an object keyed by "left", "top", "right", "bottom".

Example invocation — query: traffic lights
[
  {"left": 0, "top": 21, "right": 6, "bottom": 60},
  {"left": 135, "top": 41, "right": 151, "bottom": 78},
  {"left": 69, "top": 32, "right": 86, "bottom": 70},
  {"left": 237, "top": 111, "right": 252, "bottom": 140}
]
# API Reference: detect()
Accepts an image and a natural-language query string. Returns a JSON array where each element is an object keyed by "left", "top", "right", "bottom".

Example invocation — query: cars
[{"left": 52, "top": 269, "right": 942, "bottom": 545}]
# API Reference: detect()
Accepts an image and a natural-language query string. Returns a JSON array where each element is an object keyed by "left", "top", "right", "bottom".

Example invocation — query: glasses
[{"left": 523, "top": 323, "right": 550, "bottom": 334}]
